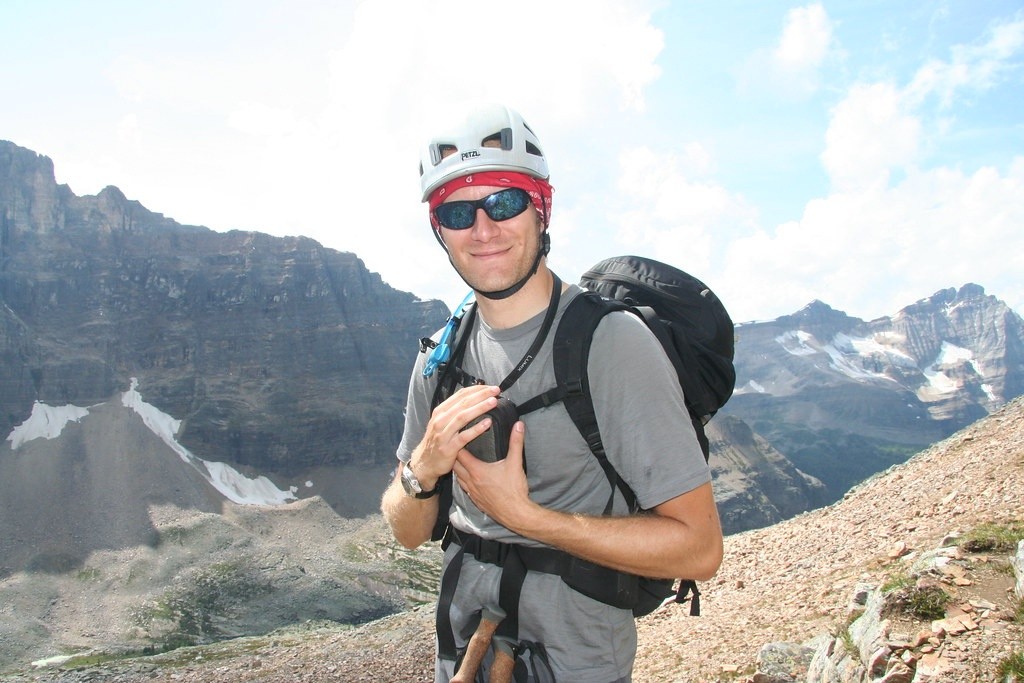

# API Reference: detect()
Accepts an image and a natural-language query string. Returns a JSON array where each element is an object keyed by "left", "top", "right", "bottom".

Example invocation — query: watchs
[{"left": 401, "top": 460, "right": 436, "bottom": 499}]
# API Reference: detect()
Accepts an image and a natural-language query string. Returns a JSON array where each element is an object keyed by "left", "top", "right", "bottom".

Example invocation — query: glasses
[{"left": 433, "top": 186, "right": 532, "bottom": 230}]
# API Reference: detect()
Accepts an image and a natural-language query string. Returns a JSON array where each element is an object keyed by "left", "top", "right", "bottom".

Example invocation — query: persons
[{"left": 383, "top": 123, "right": 721, "bottom": 683}]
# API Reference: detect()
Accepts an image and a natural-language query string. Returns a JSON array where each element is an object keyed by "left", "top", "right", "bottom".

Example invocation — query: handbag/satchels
[{"left": 460, "top": 396, "right": 527, "bottom": 474}]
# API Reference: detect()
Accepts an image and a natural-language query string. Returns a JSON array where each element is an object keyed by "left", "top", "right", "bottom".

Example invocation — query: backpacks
[{"left": 578, "top": 254, "right": 737, "bottom": 429}]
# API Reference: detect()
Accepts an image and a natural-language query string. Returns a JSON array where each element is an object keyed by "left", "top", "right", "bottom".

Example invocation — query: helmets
[{"left": 420, "top": 103, "right": 551, "bottom": 203}]
